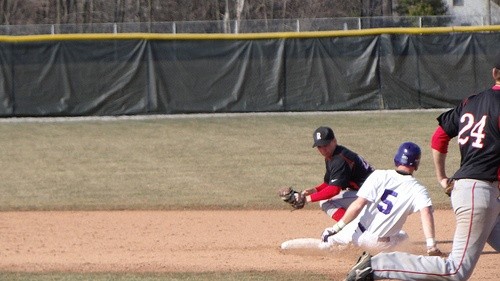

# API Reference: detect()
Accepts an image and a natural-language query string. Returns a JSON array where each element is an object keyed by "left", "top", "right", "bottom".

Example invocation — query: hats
[{"left": 312, "top": 126, "right": 334, "bottom": 148}]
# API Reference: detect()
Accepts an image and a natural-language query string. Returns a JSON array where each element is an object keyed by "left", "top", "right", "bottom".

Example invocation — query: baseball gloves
[{"left": 278, "top": 185, "right": 306, "bottom": 211}]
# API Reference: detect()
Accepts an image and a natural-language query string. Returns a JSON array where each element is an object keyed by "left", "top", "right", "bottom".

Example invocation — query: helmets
[{"left": 394, "top": 142, "right": 421, "bottom": 171}]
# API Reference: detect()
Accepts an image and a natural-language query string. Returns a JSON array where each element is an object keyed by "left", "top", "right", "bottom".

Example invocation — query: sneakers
[{"left": 344, "top": 251, "right": 373, "bottom": 281}]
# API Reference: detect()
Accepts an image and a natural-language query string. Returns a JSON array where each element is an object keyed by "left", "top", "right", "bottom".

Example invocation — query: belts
[{"left": 358, "top": 222, "right": 390, "bottom": 243}]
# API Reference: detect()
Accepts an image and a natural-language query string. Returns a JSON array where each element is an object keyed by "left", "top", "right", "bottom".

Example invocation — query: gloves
[
  {"left": 428, "top": 245, "right": 442, "bottom": 256},
  {"left": 321, "top": 224, "right": 342, "bottom": 242}
]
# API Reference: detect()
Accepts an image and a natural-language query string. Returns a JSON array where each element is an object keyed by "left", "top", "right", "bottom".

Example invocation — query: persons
[
  {"left": 293, "top": 127, "right": 376, "bottom": 222},
  {"left": 281, "top": 141, "right": 446, "bottom": 256},
  {"left": 344, "top": 60, "right": 500, "bottom": 281}
]
